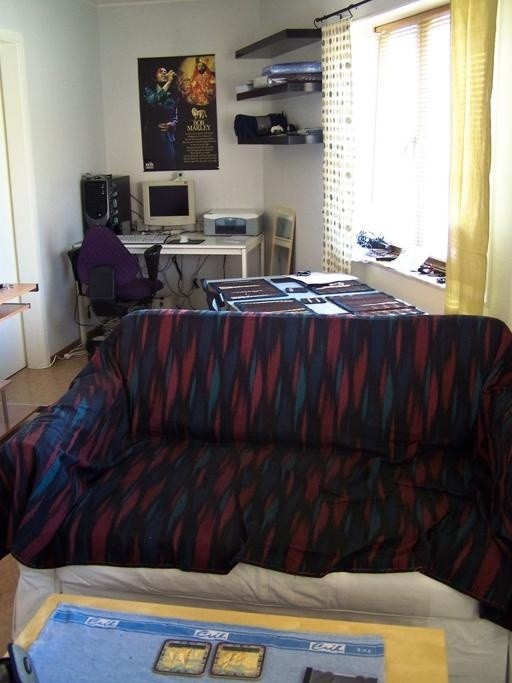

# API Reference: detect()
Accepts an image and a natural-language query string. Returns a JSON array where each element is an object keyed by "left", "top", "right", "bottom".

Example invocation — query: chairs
[{"left": 66, "top": 224, "right": 165, "bottom": 346}]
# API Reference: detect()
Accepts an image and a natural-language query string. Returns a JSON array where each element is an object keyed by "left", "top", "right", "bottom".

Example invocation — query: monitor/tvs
[{"left": 142, "top": 181, "right": 196, "bottom": 227}]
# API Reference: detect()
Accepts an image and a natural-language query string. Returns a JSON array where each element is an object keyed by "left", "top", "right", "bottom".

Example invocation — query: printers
[{"left": 202, "top": 207, "right": 264, "bottom": 236}]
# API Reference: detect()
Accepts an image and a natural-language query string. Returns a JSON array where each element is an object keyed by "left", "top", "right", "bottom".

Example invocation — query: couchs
[{"left": 0, "top": 306, "right": 512, "bottom": 635}]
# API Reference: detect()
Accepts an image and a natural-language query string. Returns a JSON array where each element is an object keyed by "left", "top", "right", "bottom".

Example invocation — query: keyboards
[{"left": 117, "top": 234, "right": 169, "bottom": 244}]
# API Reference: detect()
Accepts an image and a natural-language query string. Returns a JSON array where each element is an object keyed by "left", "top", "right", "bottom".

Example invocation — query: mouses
[{"left": 180, "top": 234, "right": 188, "bottom": 244}]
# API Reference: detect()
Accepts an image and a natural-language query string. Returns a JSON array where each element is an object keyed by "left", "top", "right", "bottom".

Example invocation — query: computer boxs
[{"left": 80, "top": 174, "right": 133, "bottom": 235}]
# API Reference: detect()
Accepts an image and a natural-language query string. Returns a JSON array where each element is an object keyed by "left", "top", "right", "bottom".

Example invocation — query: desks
[
  {"left": 72, "top": 228, "right": 266, "bottom": 278},
  {"left": 200, "top": 268, "right": 429, "bottom": 316}
]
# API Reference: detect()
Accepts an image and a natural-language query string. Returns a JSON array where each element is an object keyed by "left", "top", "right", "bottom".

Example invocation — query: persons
[
  {"left": 142, "top": 67, "right": 184, "bottom": 170},
  {"left": 184, "top": 55, "right": 215, "bottom": 107}
]
